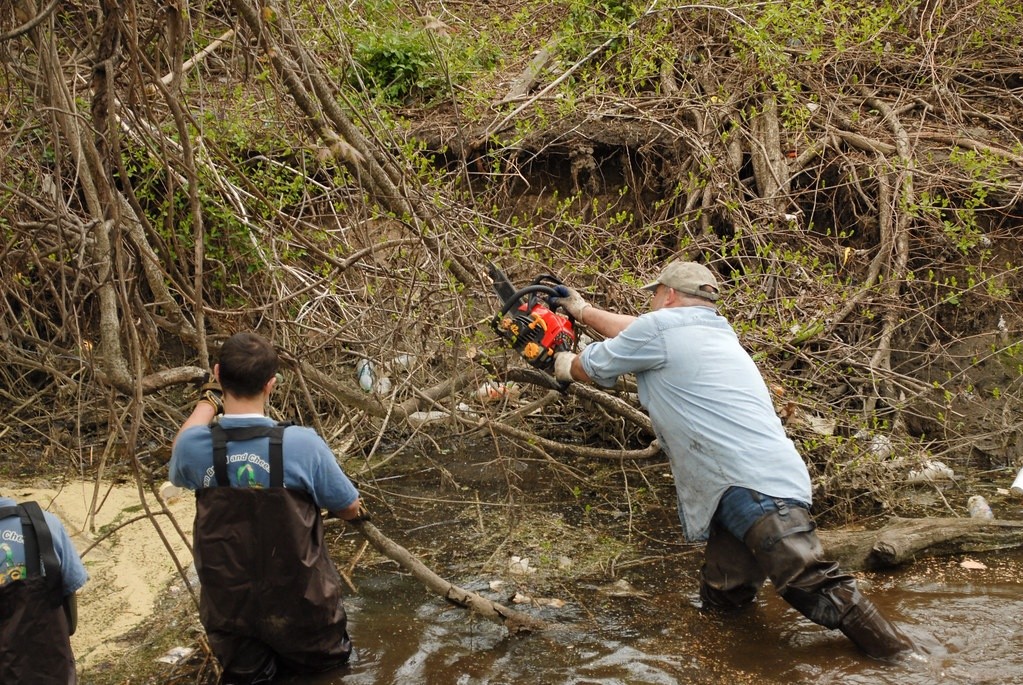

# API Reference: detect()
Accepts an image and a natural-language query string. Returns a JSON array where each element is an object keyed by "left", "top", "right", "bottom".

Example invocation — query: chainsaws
[{"left": 485, "top": 262, "right": 578, "bottom": 377}]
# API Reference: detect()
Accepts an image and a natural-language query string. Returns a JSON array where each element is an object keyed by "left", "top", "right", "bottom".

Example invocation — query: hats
[{"left": 639, "top": 262, "right": 720, "bottom": 301}]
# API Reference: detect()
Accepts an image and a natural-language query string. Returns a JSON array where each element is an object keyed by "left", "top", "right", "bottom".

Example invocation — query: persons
[
  {"left": 168, "top": 328, "right": 366, "bottom": 685},
  {"left": 540, "top": 259, "right": 916, "bottom": 658},
  {"left": 1, "top": 497, "right": 92, "bottom": 685}
]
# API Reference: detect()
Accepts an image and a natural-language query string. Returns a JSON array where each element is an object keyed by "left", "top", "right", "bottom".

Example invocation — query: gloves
[
  {"left": 554, "top": 351, "right": 577, "bottom": 382},
  {"left": 346, "top": 502, "right": 372, "bottom": 526},
  {"left": 542, "top": 284, "right": 593, "bottom": 325},
  {"left": 199, "top": 370, "right": 224, "bottom": 416}
]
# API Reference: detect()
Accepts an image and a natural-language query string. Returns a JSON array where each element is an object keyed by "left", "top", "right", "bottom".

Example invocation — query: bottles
[
  {"left": 1009, "top": 465, "right": 1023, "bottom": 497},
  {"left": 968, "top": 495, "right": 995, "bottom": 518},
  {"left": 358, "top": 346, "right": 520, "bottom": 427},
  {"left": 874, "top": 435, "right": 954, "bottom": 485}
]
